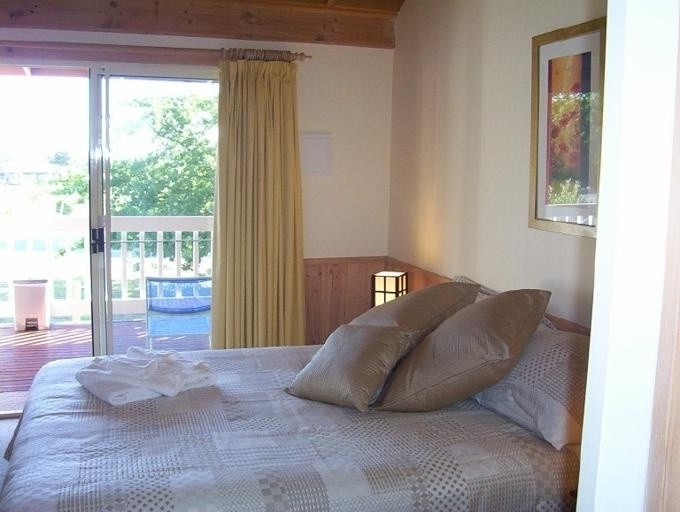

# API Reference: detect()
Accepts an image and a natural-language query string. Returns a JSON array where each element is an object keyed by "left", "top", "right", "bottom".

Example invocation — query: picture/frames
[{"left": 528, "top": 16, "right": 607, "bottom": 239}]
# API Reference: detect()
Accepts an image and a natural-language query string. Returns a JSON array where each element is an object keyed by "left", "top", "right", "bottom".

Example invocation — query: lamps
[{"left": 371, "top": 270, "right": 409, "bottom": 310}]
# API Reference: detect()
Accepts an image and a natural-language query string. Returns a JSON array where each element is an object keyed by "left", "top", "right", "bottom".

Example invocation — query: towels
[{"left": 75, "top": 345, "right": 217, "bottom": 406}]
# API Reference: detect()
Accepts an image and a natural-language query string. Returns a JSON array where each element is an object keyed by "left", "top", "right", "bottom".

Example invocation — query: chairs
[{"left": 145, "top": 274, "right": 214, "bottom": 352}]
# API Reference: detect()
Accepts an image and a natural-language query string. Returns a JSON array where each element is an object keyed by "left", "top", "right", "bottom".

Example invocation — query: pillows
[
  {"left": 357, "top": 283, "right": 478, "bottom": 354},
  {"left": 287, "top": 324, "right": 417, "bottom": 413},
  {"left": 374, "top": 289, "right": 553, "bottom": 413},
  {"left": 454, "top": 275, "right": 497, "bottom": 303},
  {"left": 474, "top": 318, "right": 589, "bottom": 449}
]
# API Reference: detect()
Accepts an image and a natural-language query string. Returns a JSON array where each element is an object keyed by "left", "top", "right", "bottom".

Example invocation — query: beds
[{"left": 1, "top": 275, "right": 587, "bottom": 512}]
